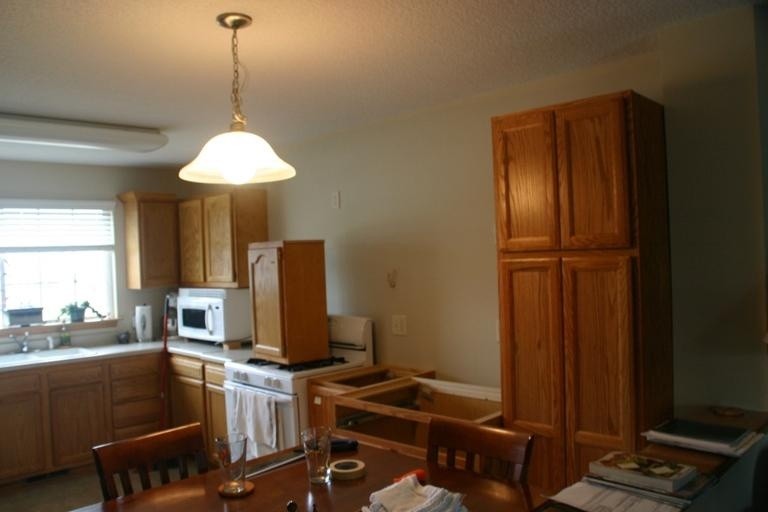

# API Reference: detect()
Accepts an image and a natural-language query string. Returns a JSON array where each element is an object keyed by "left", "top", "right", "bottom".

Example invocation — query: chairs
[
  {"left": 426, "top": 414, "right": 535, "bottom": 480},
  {"left": 91, "top": 422, "right": 208, "bottom": 502}
]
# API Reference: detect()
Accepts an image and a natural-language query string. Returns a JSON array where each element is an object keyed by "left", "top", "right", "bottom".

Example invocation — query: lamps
[{"left": 179, "top": 12, "right": 295, "bottom": 184}]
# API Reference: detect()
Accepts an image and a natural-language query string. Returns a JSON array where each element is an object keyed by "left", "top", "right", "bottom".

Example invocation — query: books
[{"left": 583, "top": 418, "right": 765, "bottom": 490}]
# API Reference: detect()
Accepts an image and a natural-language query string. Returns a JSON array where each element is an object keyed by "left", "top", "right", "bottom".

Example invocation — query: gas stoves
[{"left": 222, "top": 348, "right": 374, "bottom": 397}]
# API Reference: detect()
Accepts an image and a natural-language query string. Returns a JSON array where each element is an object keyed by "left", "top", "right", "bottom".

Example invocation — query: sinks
[
  {"left": 0, "top": 352, "right": 36, "bottom": 365},
  {"left": 35, "top": 345, "right": 90, "bottom": 359}
]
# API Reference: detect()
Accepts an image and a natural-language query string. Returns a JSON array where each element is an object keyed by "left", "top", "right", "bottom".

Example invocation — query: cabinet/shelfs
[
  {"left": 491, "top": 88, "right": 670, "bottom": 251},
  {"left": 178, "top": 188, "right": 269, "bottom": 288},
  {"left": 247, "top": 239, "right": 329, "bottom": 366},
  {"left": 497, "top": 249, "right": 673, "bottom": 492},
  {"left": 107, "top": 353, "right": 166, "bottom": 441},
  {"left": 117, "top": 190, "right": 179, "bottom": 289},
  {"left": 0, "top": 359, "right": 112, "bottom": 483},
  {"left": 168, "top": 353, "right": 228, "bottom": 452}
]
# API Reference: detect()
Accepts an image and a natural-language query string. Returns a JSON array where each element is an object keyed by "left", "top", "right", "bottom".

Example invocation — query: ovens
[{"left": 221, "top": 380, "right": 312, "bottom": 466}]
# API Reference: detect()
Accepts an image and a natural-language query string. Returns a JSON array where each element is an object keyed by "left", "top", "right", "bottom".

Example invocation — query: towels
[
  {"left": 231, "top": 386, "right": 284, "bottom": 456},
  {"left": 360, "top": 475, "right": 468, "bottom": 512}
]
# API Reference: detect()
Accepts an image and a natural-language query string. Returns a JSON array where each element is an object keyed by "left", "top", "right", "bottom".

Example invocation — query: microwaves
[{"left": 175, "top": 287, "right": 251, "bottom": 345}]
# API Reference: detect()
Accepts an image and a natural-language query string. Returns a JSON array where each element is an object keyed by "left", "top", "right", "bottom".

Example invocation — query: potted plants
[{"left": 58, "top": 302, "right": 84, "bottom": 323}]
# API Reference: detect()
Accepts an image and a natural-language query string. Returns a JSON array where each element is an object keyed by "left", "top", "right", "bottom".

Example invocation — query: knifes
[{"left": 230, "top": 437, "right": 357, "bottom": 482}]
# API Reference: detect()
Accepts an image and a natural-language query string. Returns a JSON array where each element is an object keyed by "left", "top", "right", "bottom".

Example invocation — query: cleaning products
[{"left": 60, "top": 326, "right": 72, "bottom": 345}]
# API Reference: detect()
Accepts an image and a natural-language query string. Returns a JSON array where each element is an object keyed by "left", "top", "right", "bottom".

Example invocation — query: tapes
[{"left": 330, "top": 459, "right": 366, "bottom": 480}]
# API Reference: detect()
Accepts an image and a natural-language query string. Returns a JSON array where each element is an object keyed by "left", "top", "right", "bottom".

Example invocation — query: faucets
[{"left": 8, "top": 330, "right": 29, "bottom": 352}]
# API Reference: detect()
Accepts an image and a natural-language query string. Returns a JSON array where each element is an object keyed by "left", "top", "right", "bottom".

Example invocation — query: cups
[
  {"left": 211, "top": 434, "right": 247, "bottom": 493},
  {"left": 298, "top": 425, "right": 330, "bottom": 487}
]
